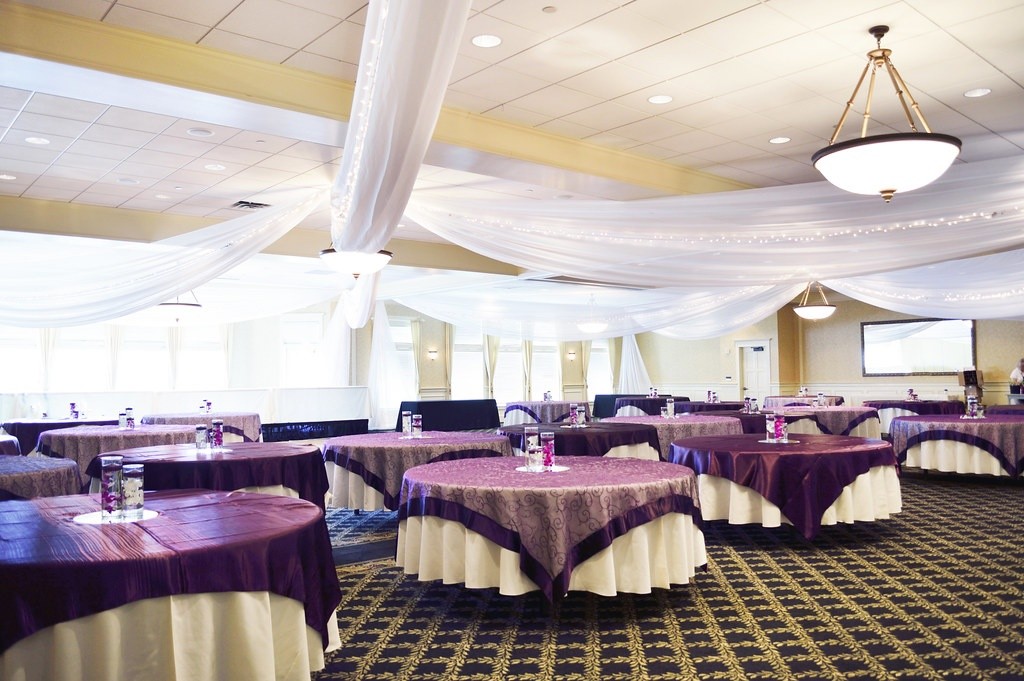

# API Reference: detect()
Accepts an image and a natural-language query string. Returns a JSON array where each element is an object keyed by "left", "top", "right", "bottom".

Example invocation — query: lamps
[
  {"left": 428, "top": 347, "right": 438, "bottom": 361},
  {"left": 567, "top": 351, "right": 577, "bottom": 362},
  {"left": 793, "top": 280, "right": 838, "bottom": 323},
  {"left": 158, "top": 290, "right": 202, "bottom": 324},
  {"left": 810, "top": 24, "right": 963, "bottom": 203},
  {"left": 319, "top": 238, "right": 395, "bottom": 280}
]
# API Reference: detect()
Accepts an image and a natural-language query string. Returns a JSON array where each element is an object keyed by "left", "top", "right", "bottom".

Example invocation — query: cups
[
  {"left": 119, "top": 408, "right": 133, "bottom": 430},
  {"left": 708, "top": 390, "right": 717, "bottom": 403},
  {"left": 799, "top": 386, "right": 807, "bottom": 396},
  {"left": 402, "top": 411, "right": 422, "bottom": 436},
  {"left": 121, "top": 465, "right": 145, "bottom": 520},
  {"left": 907, "top": 388, "right": 917, "bottom": 401},
  {"left": 70, "top": 402, "right": 78, "bottom": 419},
  {"left": 744, "top": 397, "right": 758, "bottom": 414},
  {"left": 101, "top": 455, "right": 124, "bottom": 521},
  {"left": 650, "top": 387, "right": 657, "bottom": 397},
  {"left": 196, "top": 419, "right": 223, "bottom": 449},
  {"left": 544, "top": 390, "right": 550, "bottom": 403},
  {"left": 813, "top": 392, "right": 824, "bottom": 408},
  {"left": 570, "top": 404, "right": 585, "bottom": 425},
  {"left": 199, "top": 399, "right": 211, "bottom": 413},
  {"left": 967, "top": 395, "right": 983, "bottom": 418},
  {"left": 782, "top": 423, "right": 787, "bottom": 441},
  {"left": 525, "top": 427, "right": 554, "bottom": 472},
  {"left": 660, "top": 399, "right": 674, "bottom": 418},
  {"left": 766, "top": 414, "right": 784, "bottom": 443}
]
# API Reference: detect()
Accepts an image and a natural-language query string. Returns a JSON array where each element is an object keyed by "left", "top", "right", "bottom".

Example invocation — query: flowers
[{"left": 70, "top": 386, "right": 989, "bottom": 515}]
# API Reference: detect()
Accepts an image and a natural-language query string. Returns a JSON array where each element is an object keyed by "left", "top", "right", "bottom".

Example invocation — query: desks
[{"left": 0, "top": 393, "right": 1021, "bottom": 681}]
